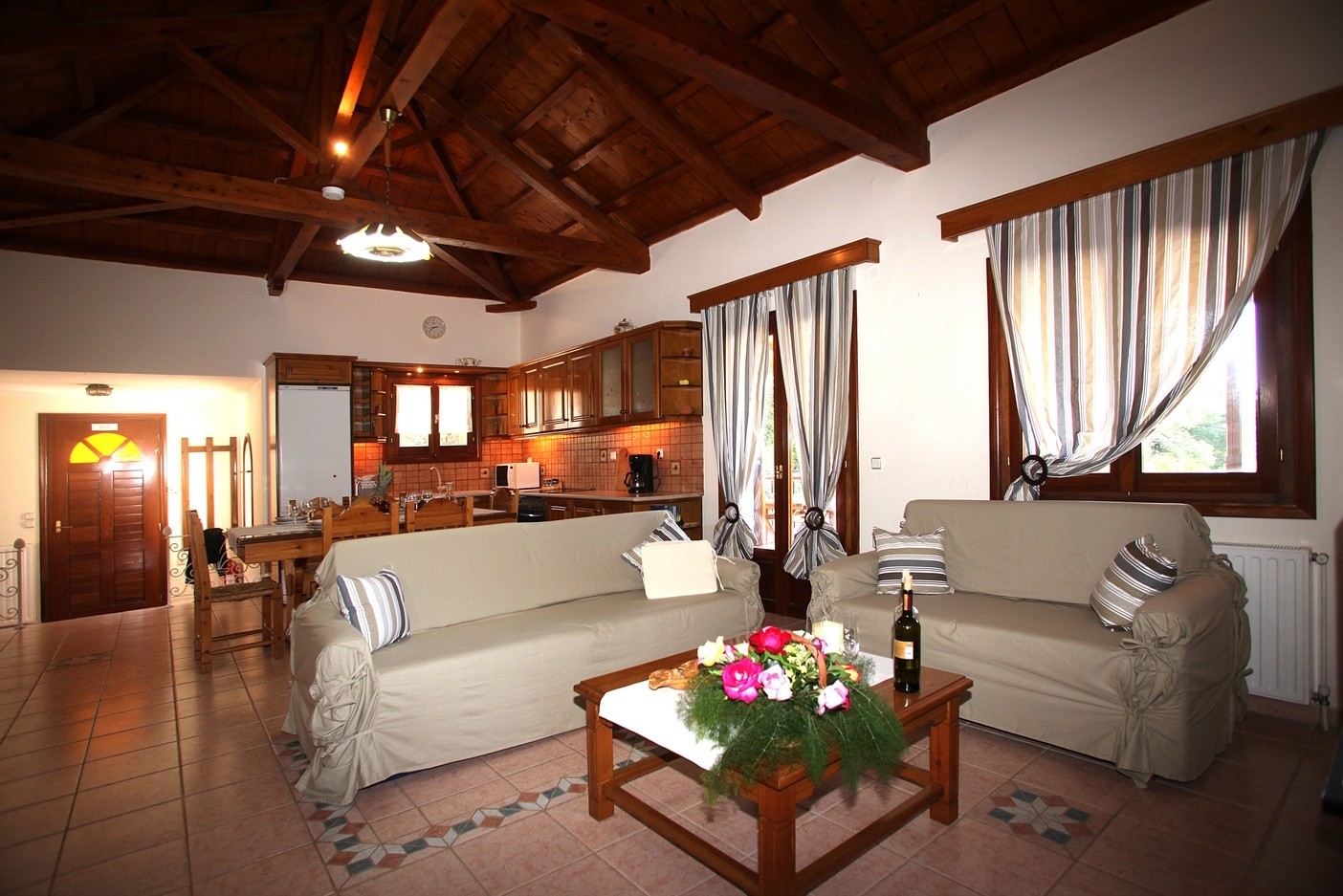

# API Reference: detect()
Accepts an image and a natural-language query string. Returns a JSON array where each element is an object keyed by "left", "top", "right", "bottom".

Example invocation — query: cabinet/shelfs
[{"left": 505, "top": 320, "right": 702, "bottom": 440}]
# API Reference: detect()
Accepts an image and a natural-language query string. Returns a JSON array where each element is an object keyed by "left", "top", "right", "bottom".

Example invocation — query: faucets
[{"left": 429, "top": 466, "right": 447, "bottom": 495}]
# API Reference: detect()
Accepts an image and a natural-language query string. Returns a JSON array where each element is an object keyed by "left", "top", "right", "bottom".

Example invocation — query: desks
[{"left": 228, "top": 504, "right": 517, "bottom": 650}]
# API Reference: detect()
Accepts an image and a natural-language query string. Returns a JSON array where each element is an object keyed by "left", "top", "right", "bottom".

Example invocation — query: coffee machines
[{"left": 624, "top": 454, "right": 653, "bottom": 493}]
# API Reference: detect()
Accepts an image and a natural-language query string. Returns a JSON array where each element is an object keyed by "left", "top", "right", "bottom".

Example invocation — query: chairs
[
  {"left": 296, "top": 496, "right": 402, "bottom": 603},
  {"left": 490, "top": 485, "right": 521, "bottom": 524},
  {"left": 183, "top": 513, "right": 284, "bottom": 675},
  {"left": 404, "top": 495, "right": 474, "bottom": 531}
]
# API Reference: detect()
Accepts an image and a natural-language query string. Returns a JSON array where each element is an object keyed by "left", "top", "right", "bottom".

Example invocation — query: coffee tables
[{"left": 574, "top": 637, "right": 979, "bottom": 894}]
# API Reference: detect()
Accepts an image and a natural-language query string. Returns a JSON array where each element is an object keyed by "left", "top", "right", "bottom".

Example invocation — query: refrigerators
[{"left": 276, "top": 384, "right": 353, "bottom": 519}]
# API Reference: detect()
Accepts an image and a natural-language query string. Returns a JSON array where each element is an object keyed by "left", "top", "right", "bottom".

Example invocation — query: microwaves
[{"left": 495, "top": 462, "right": 540, "bottom": 489}]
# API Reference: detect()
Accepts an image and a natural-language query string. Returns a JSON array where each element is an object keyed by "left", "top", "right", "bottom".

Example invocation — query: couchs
[
  {"left": 281, "top": 511, "right": 763, "bottom": 809},
  {"left": 810, "top": 499, "right": 1252, "bottom": 792}
]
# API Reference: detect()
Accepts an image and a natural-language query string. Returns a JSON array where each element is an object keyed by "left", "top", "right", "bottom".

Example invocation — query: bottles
[{"left": 893, "top": 570, "right": 921, "bottom": 693}]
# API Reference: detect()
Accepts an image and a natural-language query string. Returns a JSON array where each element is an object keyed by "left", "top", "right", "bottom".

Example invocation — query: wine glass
[{"left": 289, "top": 497, "right": 332, "bottom": 529}]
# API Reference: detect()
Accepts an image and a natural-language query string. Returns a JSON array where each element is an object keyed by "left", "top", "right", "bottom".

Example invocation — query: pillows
[
  {"left": 872, "top": 526, "right": 953, "bottom": 595},
  {"left": 1087, "top": 531, "right": 1180, "bottom": 631},
  {"left": 334, "top": 567, "right": 412, "bottom": 653},
  {"left": 621, "top": 513, "right": 696, "bottom": 571}
]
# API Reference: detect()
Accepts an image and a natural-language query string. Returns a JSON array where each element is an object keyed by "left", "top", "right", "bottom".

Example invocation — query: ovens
[{"left": 517, "top": 496, "right": 546, "bottom": 522}]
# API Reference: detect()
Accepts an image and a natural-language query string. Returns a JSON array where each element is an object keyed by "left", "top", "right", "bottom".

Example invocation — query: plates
[{"left": 273, "top": 516, "right": 307, "bottom": 524}]
